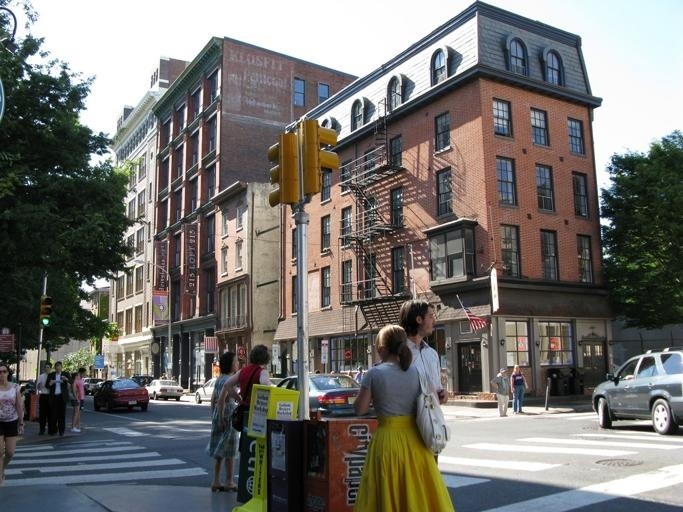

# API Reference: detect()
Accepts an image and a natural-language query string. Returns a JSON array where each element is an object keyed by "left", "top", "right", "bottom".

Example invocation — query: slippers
[
  {"left": 223, "top": 486, "right": 238, "bottom": 491},
  {"left": 212, "top": 486, "right": 224, "bottom": 491}
]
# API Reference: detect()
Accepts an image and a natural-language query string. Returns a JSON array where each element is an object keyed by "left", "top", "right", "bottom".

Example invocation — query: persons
[
  {"left": 45, "top": 361, "right": 73, "bottom": 436},
  {"left": 224, "top": 343, "right": 275, "bottom": 503},
  {"left": 205, "top": 352, "right": 239, "bottom": 492},
  {"left": 0, "top": 364, "right": 24, "bottom": 487},
  {"left": 72, "top": 368, "right": 86, "bottom": 428},
  {"left": 71, "top": 372, "right": 81, "bottom": 433},
  {"left": 37, "top": 362, "right": 58, "bottom": 435},
  {"left": 352, "top": 325, "right": 456, "bottom": 512},
  {"left": 491, "top": 367, "right": 510, "bottom": 417},
  {"left": 398, "top": 299, "right": 448, "bottom": 470},
  {"left": 510, "top": 365, "right": 529, "bottom": 415}
]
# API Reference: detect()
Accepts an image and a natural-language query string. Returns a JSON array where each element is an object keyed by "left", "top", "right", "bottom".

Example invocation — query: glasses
[
  {"left": 0, "top": 370, "right": 8, "bottom": 374},
  {"left": 43, "top": 366, "right": 50, "bottom": 368}
]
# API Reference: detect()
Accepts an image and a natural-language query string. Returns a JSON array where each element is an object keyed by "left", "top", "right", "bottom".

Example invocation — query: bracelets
[
  {"left": 19, "top": 424, "right": 23, "bottom": 425},
  {"left": 235, "top": 398, "right": 241, "bottom": 403}
]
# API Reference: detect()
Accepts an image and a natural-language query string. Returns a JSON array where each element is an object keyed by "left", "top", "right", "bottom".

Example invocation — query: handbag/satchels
[
  {"left": 68, "top": 391, "right": 77, "bottom": 400},
  {"left": 413, "top": 367, "right": 450, "bottom": 455},
  {"left": 231, "top": 366, "right": 261, "bottom": 431}
]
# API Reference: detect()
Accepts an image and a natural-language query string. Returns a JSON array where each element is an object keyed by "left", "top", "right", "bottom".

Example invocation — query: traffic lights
[
  {"left": 268, "top": 133, "right": 300, "bottom": 207},
  {"left": 300, "top": 118, "right": 340, "bottom": 194},
  {"left": 41, "top": 296, "right": 53, "bottom": 327}
]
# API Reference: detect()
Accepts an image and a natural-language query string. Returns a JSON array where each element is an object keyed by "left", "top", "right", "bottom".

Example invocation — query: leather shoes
[
  {"left": 52, "top": 433, "right": 57, "bottom": 436},
  {"left": 59, "top": 432, "right": 63, "bottom": 435}
]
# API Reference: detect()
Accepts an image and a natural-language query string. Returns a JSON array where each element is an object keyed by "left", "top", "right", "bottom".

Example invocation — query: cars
[
  {"left": 196, "top": 379, "right": 216, "bottom": 403},
  {"left": 592, "top": 351, "right": 682, "bottom": 435},
  {"left": 277, "top": 374, "right": 375, "bottom": 417},
  {"left": 94, "top": 379, "right": 149, "bottom": 411},
  {"left": 83, "top": 378, "right": 105, "bottom": 396},
  {"left": 146, "top": 380, "right": 183, "bottom": 401}
]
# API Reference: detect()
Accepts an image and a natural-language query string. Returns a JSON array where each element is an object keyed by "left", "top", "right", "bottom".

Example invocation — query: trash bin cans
[
  {"left": 567, "top": 367, "right": 583, "bottom": 395},
  {"left": 304, "top": 419, "right": 380, "bottom": 512},
  {"left": 546, "top": 368, "right": 565, "bottom": 396},
  {"left": 267, "top": 418, "right": 302, "bottom": 512},
  {"left": 24, "top": 393, "right": 35, "bottom": 422}
]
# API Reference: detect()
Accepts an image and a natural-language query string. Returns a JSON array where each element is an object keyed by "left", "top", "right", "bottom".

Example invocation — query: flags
[{"left": 455, "top": 294, "right": 489, "bottom": 336}]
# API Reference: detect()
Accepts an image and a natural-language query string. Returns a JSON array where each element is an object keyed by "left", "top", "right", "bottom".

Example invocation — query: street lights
[{"left": 134, "top": 259, "right": 172, "bottom": 378}]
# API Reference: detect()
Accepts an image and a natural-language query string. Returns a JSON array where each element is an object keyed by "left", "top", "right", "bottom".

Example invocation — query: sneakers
[{"left": 71, "top": 427, "right": 81, "bottom": 432}]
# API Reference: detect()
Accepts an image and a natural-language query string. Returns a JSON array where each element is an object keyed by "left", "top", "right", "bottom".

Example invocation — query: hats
[{"left": 500, "top": 368, "right": 508, "bottom": 373}]
